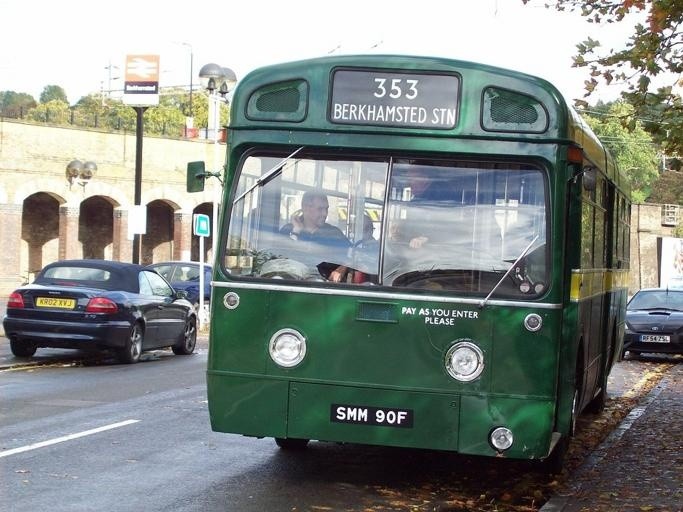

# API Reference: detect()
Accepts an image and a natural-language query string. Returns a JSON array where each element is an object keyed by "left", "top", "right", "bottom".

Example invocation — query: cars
[
  {"left": 622, "top": 288, "right": 683, "bottom": 359},
  {"left": 3, "top": 261, "right": 212, "bottom": 364}
]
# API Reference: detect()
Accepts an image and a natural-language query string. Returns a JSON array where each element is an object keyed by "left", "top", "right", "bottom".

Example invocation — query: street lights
[{"left": 199, "top": 62, "right": 237, "bottom": 140}]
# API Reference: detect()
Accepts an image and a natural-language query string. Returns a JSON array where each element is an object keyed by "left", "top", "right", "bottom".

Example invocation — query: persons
[
  {"left": 278, "top": 189, "right": 354, "bottom": 283},
  {"left": 390, "top": 219, "right": 404, "bottom": 244},
  {"left": 405, "top": 159, "right": 461, "bottom": 250},
  {"left": 354, "top": 216, "right": 375, "bottom": 240}
]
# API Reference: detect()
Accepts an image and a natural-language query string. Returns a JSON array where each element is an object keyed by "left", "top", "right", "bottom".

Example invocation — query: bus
[{"left": 185, "top": 53, "right": 632, "bottom": 466}]
